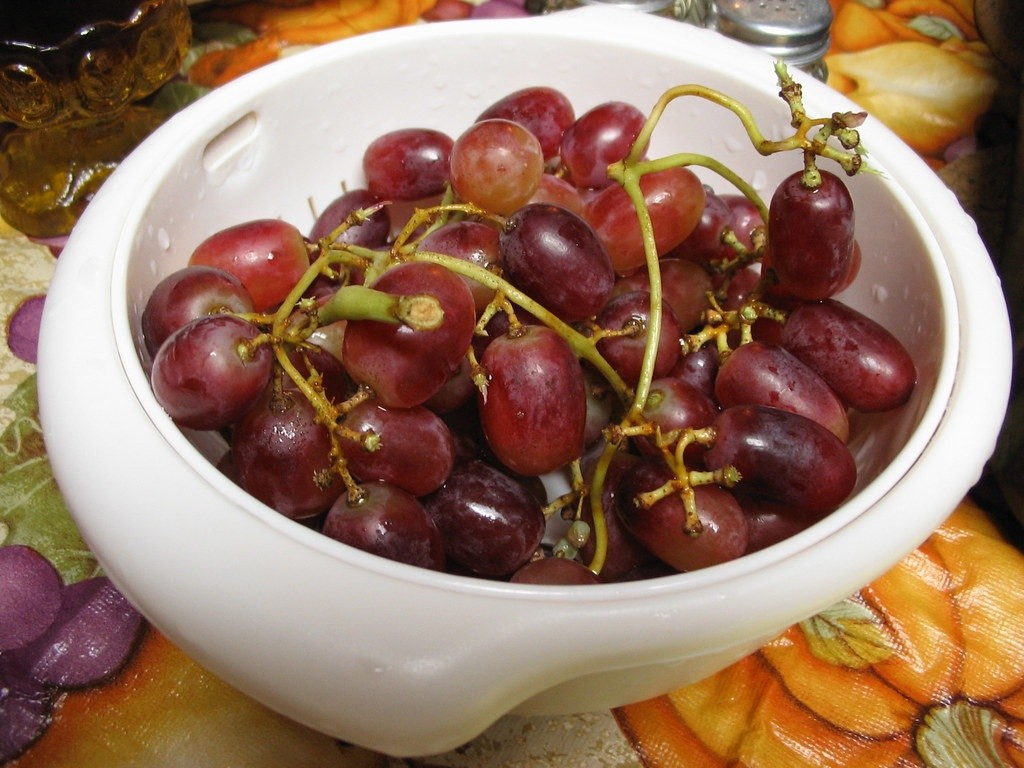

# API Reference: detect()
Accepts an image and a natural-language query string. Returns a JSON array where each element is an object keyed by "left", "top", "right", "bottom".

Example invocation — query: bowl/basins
[{"left": 39, "top": 5, "right": 1012, "bottom": 757}]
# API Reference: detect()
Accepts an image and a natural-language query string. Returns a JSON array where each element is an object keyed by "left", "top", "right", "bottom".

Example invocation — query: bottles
[{"left": 710, "top": 0, "right": 832, "bottom": 82}]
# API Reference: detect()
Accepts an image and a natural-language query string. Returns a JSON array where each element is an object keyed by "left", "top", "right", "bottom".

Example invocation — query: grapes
[{"left": 138, "top": 84, "right": 917, "bottom": 585}]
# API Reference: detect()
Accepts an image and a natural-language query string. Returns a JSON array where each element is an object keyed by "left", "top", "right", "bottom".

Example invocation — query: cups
[
  {"left": 0, "top": 0, "right": 191, "bottom": 237},
  {"left": 543, "top": 0, "right": 676, "bottom": 20}
]
